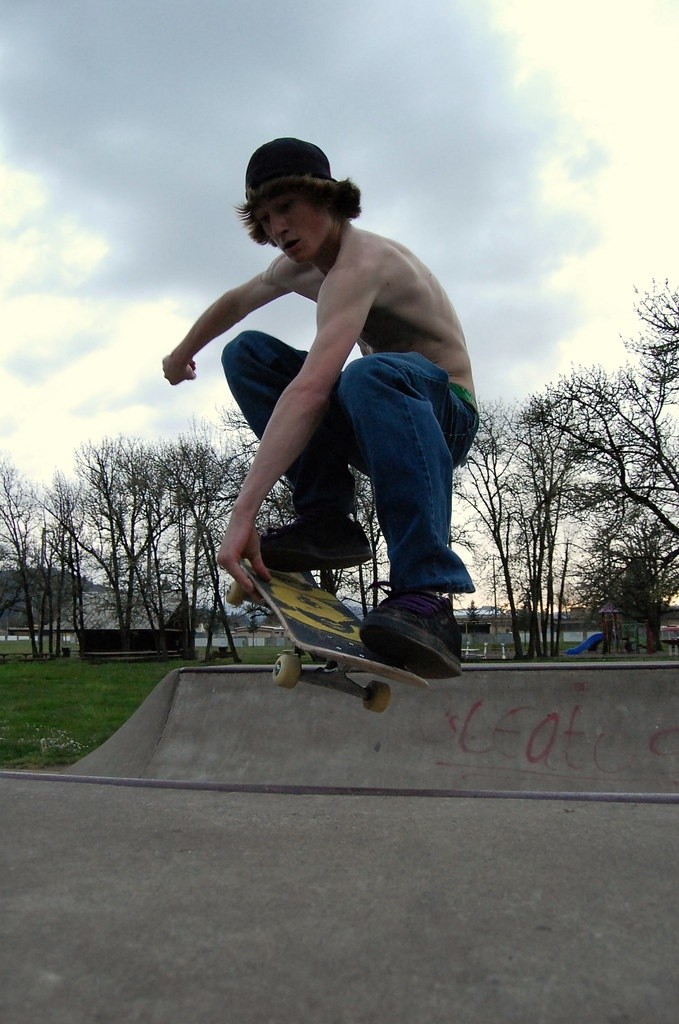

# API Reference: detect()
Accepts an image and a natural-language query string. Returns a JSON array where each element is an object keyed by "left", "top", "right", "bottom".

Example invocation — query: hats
[{"left": 245, "top": 138, "right": 339, "bottom": 200}]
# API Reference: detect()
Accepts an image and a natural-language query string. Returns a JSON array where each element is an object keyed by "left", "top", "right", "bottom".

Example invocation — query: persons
[{"left": 158, "top": 137, "right": 480, "bottom": 672}]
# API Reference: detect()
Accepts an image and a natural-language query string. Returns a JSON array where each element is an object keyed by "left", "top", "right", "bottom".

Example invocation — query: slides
[
  {"left": 638, "top": 636, "right": 669, "bottom": 652},
  {"left": 565, "top": 633, "right": 605, "bottom": 654}
]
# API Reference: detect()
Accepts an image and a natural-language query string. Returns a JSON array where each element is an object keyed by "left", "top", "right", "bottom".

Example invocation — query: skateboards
[{"left": 224, "top": 553, "right": 430, "bottom": 714}]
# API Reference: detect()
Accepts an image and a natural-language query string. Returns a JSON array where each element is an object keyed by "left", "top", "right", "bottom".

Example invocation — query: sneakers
[
  {"left": 360, "top": 591, "right": 461, "bottom": 678},
  {"left": 262, "top": 518, "right": 372, "bottom": 571}
]
